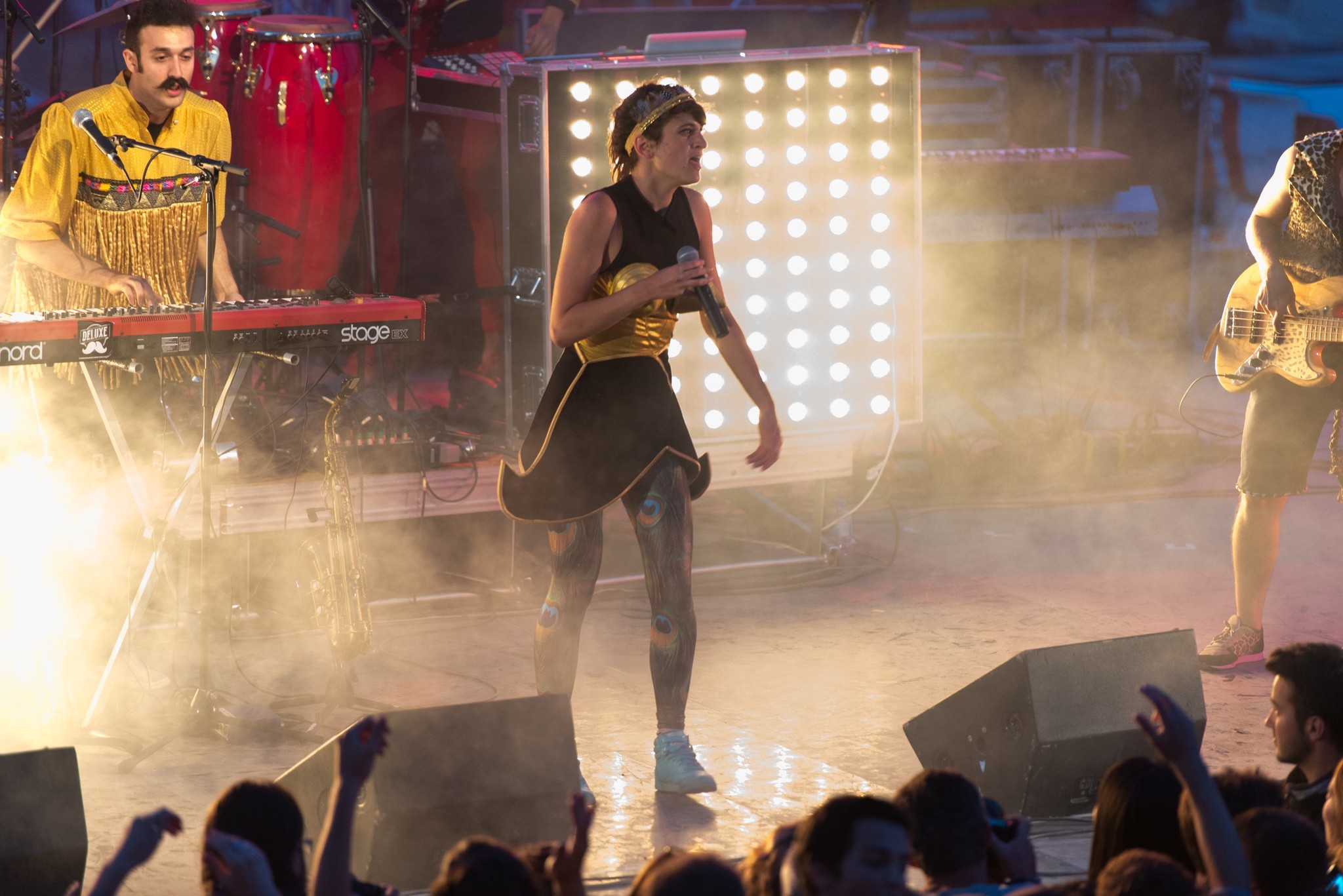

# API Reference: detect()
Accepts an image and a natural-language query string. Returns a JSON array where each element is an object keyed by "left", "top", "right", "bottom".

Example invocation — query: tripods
[{"left": 110, "top": 134, "right": 331, "bottom": 775}]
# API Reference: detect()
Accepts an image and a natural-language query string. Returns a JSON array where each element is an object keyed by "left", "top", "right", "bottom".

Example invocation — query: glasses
[{"left": 293, "top": 838, "right": 313, "bottom": 863}]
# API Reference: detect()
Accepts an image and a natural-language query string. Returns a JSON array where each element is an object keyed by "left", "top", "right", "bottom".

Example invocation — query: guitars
[{"left": 1214, "top": 261, "right": 1343, "bottom": 393}]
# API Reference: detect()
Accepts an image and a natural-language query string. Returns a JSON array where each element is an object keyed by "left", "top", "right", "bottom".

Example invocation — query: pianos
[{"left": 921, "top": 143, "right": 1163, "bottom": 399}]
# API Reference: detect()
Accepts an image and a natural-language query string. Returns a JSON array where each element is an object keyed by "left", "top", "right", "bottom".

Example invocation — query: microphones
[
  {"left": 71, "top": 108, "right": 125, "bottom": 170},
  {"left": 676, "top": 245, "right": 732, "bottom": 339}
]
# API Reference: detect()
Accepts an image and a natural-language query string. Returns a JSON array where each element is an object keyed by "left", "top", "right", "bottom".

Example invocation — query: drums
[{"left": 185, "top": 0, "right": 370, "bottom": 300}]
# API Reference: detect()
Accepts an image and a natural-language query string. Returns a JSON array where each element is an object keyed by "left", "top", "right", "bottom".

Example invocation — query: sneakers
[
  {"left": 654, "top": 731, "right": 717, "bottom": 793},
  {"left": 1198, "top": 615, "right": 1264, "bottom": 669},
  {"left": 576, "top": 772, "right": 596, "bottom": 804}
]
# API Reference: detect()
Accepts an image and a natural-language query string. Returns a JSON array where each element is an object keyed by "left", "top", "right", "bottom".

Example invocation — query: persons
[
  {"left": 0, "top": 0, "right": 248, "bottom": 461},
  {"left": 0, "top": 642, "right": 1343, "bottom": 896},
  {"left": 499, "top": 83, "right": 781, "bottom": 795},
  {"left": 1196, "top": 131, "right": 1343, "bottom": 669},
  {"left": 0, "top": 57, "right": 19, "bottom": 85},
  {"left": 398, "top": 0, "right": 579, "bottom": 384}
]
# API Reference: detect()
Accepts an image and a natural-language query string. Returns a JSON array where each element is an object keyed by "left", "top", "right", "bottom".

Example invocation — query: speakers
[
  {"left": 269, "top": 692, "right": 583, "bottom": 893},
  {"left": 0, "top": 745, "right": 88, "bottom": 896},
  {"left": 900, "top": 627, "right": 1207, "bottom": 825}
]
